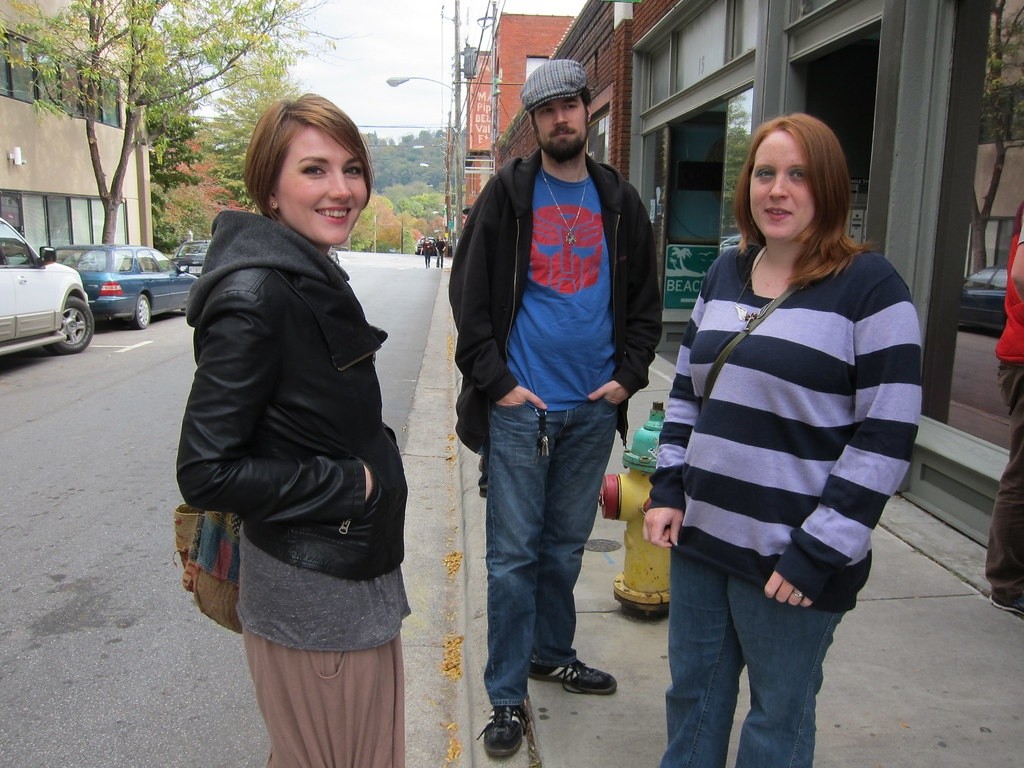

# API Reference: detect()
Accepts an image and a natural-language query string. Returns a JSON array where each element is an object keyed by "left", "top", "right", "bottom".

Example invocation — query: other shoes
[{"left": 989, "top": 594, "right": 1024, "bottom": 613}]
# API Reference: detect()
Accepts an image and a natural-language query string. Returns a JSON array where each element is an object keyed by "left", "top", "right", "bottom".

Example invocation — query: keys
[{"left": 536, "top": 435, "right": 550, "bottom": 456}]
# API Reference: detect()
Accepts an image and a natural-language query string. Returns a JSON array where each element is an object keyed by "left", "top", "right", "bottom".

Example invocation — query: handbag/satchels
[{"left": 174, "top": 505, "right": 243, "bottom": 634}]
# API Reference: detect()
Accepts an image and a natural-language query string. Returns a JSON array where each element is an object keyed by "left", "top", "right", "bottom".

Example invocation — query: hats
[{"left": 520, "top": 59, "right": 587, "bottom": 110}]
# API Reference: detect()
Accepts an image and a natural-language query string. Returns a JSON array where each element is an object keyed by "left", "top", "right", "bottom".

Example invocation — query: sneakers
[
  {"left": 528, "top": 661, "right": 616, "bottom": 694},
  {"left": 477, "top": 705, "right": 530, "bottom": 757}
]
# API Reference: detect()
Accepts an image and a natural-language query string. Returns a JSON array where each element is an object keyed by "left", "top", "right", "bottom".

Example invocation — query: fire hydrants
[{"left": 599, "top": 401, "right": 671, "bottom": 619}]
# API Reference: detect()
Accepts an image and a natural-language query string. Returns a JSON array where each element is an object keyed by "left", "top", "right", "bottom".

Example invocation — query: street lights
[
  {"left": 386, "top": 76, "right": 462, "bottom": 248},
  {"left": 418, "top": 163, "right": 452, "bottom": 258}
]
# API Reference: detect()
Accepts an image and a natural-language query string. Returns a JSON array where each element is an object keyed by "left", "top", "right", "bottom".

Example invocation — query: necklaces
[
  {"left": 734, "top": 249, "right": 779, "bottom": 331},
  {"left": 539, "top": 166, "right": 589, "bottom": 244}
]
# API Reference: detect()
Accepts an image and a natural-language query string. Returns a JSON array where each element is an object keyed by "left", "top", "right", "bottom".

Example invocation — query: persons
[
  {"left": 420, "top": 236, "right": 433, "bottom": 269},
  {"left": 641, "top": 112, "right": 924, "bottom": 768},
  {"left": 446, "top": 56, "right": 665, "bottom": 758},
  {"left": 176, "top": 93, "right": 415, "bottom": 768},
  {"left": 435, "top": 235, "right": 446, "bottom": 269},
  {"left": 983, "top": 194, "right": 1024, "bottom": 617}
]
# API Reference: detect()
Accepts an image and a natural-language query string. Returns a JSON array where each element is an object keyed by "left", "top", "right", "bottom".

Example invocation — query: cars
[
  {"left": 719, "top": 235, "right": 741, "bottom": 256},
  {"left": 171, "top": 238, "right": 213, "bottom": 278},
  {"left": 414, "top": 237, "right": 437, "bottom": 256},
  {"left": 958, "top": 266, "right": 1009, "bottom": 333},
  {"left": 54, "top": 243, "right": 199, "bottom": 330}
]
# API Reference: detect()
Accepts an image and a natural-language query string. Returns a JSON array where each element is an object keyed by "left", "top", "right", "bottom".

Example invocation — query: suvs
[{"left": 0, "top": 217, "right": 95, "bottom": 357}]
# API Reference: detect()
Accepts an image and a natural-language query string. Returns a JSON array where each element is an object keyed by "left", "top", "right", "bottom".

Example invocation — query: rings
[{"left": 791, "top": 589, "right": 803, "bottom": 600}]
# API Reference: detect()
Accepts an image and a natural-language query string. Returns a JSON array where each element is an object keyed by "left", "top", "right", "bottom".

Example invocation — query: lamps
[{"left": 7, "top": 146, "right": 22, "bottom": 166}]
